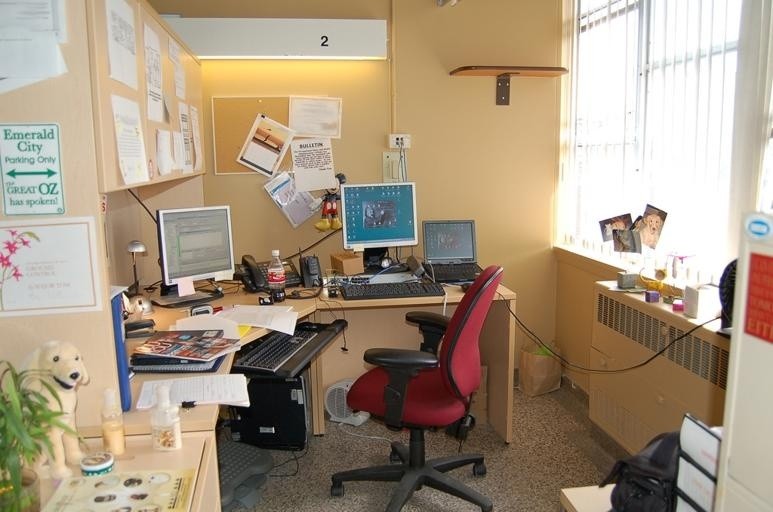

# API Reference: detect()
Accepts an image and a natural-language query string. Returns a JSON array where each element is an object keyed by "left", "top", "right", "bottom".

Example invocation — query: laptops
[{"left": 422, "top": 220, "right": 484, "bottom": 282}]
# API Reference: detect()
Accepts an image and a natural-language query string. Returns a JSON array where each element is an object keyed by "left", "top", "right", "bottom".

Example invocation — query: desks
[{"left": 561, "top": 486, "right": 625, "bottom": 512}]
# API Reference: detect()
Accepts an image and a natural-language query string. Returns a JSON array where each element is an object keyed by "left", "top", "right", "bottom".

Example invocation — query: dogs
[{"left": 638, "top": 214, "right": 663, "bottom": 248}]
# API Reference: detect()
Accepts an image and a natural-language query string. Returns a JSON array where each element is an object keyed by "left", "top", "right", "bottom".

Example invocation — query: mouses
[
  {"left": 297, "top": 322, "right": 318, "bottom": 330},
  {"left": 462, "top": 283, "right": 472, "bottom": 293}
]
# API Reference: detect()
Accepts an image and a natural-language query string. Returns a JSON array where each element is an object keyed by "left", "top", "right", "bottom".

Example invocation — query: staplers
[{"left": 125, "top": 319, "right": 156, "bottom": 337}]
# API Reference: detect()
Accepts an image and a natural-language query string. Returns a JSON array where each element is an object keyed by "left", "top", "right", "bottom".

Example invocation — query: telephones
[{"left": 235, "top": 255, "right": 302, "bottom": 292}]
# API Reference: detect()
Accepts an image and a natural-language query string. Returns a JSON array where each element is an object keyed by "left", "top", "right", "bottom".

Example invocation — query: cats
[{"left": 604, "top": 221, "right": 625, "bottom": 236}]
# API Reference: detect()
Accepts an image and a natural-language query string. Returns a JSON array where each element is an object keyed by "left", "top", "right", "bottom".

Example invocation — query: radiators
[{"left": 585, "top": 271, "right": 730, "bottom": 456}]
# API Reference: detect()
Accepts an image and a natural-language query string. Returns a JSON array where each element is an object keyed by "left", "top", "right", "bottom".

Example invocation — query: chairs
[{"left": 332, "top": 263, "right": 504, "bottom": 512}]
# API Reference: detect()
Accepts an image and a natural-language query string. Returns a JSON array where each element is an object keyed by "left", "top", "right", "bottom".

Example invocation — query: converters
[{"left": 407, "top": 255, "right": 425, "bottom": 277}]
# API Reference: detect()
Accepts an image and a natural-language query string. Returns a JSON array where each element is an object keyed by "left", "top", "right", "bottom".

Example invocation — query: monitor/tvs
[
  {"left": 340, "top": 181, "right": 418, "bottom": 274},
  {"left": 150, "top": 205, "right": 235, "bottom": 307}
]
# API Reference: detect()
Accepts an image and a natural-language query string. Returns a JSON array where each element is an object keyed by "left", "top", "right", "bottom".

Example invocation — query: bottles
[
  {"left": 149, "top": 384, "right": 182, "bottom": 451},
  {"left": 101, "top": 390, "right": 127, "bottom": 456},
  {"left": 267, "top": 250, "right": 285, "bottom": 303}
]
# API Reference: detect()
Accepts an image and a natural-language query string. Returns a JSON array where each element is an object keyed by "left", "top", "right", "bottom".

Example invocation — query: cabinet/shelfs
[
  {"left": 190, "top": 440, "right": 229, "bottom": 509},
  {"left": 1, "top": 0, "right": 207, "bottom": 192}
]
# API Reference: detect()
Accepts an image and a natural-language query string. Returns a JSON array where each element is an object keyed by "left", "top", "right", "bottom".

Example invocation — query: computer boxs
[{"left": 228, "top": 367, "right": 311, "bottom": 451}]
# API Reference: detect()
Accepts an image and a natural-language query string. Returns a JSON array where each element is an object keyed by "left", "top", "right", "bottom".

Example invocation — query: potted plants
[{"left": 0, "top": 360, "right": 90, "bottom": 512}]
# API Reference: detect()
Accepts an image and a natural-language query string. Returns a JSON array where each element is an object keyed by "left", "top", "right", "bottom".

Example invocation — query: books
[{"left": 130, "top": 329, "right": 241, "bottom": 374}]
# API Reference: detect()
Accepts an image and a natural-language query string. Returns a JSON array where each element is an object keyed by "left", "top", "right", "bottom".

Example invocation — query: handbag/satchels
[{"left": 597, "top": 430, "right": 681, "bottom": 511}]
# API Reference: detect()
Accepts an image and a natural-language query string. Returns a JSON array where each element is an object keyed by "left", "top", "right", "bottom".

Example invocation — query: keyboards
[
  {"left": 233, "top": 328, "right": 318, "bottom": 373},
  {"left": 340, "top": 282, "right": 446, "bottom": 301}
]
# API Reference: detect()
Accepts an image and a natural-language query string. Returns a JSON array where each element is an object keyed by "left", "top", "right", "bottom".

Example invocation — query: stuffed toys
[{"left": 20, "top": 340, "right": 90, "bottom": 481}]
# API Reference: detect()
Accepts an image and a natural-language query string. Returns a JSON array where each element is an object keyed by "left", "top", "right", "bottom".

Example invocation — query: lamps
[
  {"left": 160, "top": 14, "right": 388, "bottom": 60},
  {"left": 126, "top": 240, "right": 146, "bottom": 298}
]
